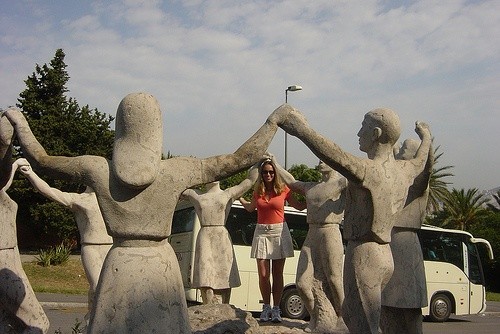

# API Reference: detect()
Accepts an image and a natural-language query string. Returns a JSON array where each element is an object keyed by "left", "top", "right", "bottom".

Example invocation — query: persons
[
  {"left": 0, "top": 92, "right": 294, "bottom": 334},
  {"left": 239, "top": 158, "right": 307, "bottom": 323},
  {"left": 280, "top": 103, "right": 434, "bottom": 334}
]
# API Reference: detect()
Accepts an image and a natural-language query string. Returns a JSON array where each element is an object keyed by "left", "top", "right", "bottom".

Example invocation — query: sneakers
[
  {"left": 271, "top": 309, "right": 282, "bottom": 322},
  {"left": 260, "top": 305, "right": 271, "bottom": 321}
]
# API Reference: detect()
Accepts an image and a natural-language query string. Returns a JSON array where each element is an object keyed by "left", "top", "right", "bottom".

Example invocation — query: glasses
[{"left": 262, "top": 170, "right": 275, "bottom": 174}]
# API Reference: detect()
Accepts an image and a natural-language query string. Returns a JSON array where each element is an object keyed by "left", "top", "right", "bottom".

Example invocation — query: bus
[{"left": 168, "top": 195, "right": 495, "bottom": 323}]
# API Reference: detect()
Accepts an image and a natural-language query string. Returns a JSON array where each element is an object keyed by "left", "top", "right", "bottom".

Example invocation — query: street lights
[{"left": 284, "top": 83, "right": 303, "bottom": 170}]
